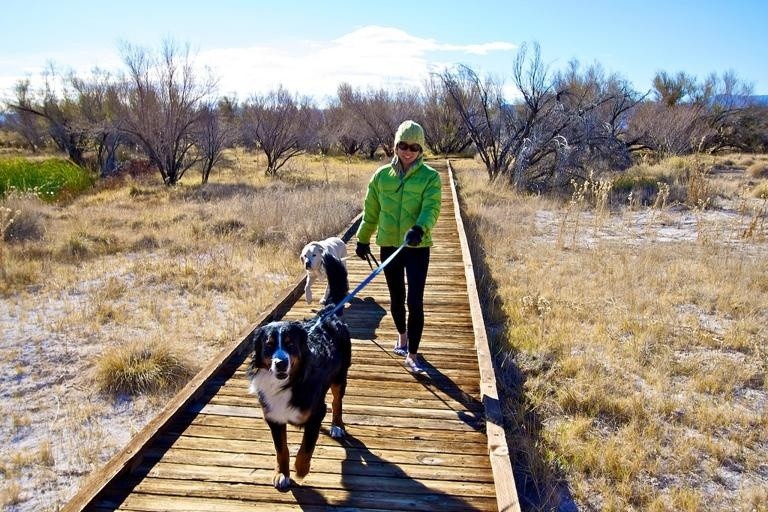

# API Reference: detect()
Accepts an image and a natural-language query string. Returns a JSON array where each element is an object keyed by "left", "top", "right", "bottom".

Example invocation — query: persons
[{"left": 354, "top": 120, "right": 442, "bottom": 375}]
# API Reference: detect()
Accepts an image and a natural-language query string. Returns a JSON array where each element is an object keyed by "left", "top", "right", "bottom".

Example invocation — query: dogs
[
  {"left": 246, "top": 253, "right": 351, "bottom": 490},
  {"left": 300, "top": 237, "right": 347, "bottom": 305}
]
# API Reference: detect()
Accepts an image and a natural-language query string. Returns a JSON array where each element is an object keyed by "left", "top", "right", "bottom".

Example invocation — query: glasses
[{"left": 399, "top": 141, "right": 419, "bottom": 153}]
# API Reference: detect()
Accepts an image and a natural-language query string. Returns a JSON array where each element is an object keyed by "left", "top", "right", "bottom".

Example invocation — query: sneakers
[
  {"left": 405, "top": 357, "right": 429, "bottom": 374},
  {"left": 394, "top": 339, "right": 407, "bottom": 356}
]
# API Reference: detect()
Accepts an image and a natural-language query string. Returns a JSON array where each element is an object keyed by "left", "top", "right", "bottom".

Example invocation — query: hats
[{"left": 393, "top": 120, "right": 424, "bottom": 152}]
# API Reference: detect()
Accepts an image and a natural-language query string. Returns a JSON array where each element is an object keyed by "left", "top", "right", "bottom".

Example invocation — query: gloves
[
  {"left": 356, "top": 242, "right": 371, "bottom": 261},
  {"left": 406, "top": 225, "right": 424, "bottom": 247}
]
